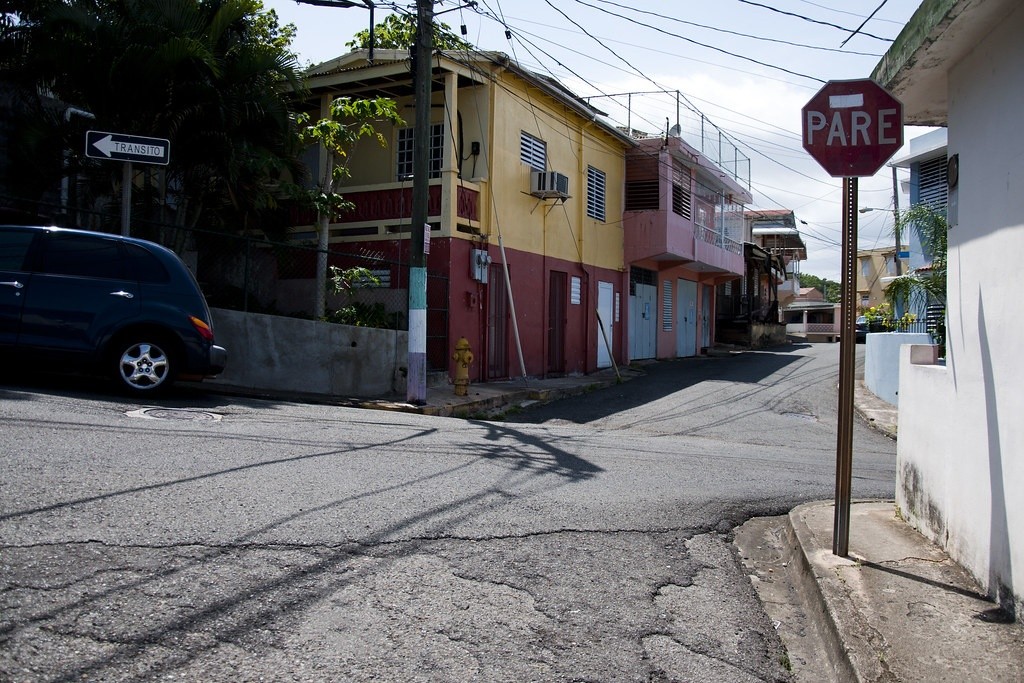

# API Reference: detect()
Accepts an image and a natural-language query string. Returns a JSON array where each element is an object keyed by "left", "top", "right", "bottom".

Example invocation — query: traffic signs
[{"left": 86, "top": 131, "right": 170, "bottom": 166}]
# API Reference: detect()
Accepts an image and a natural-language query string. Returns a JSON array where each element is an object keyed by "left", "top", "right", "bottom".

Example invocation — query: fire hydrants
[{"left": 452, "top": 336, "right": 474, "bottom": 396}]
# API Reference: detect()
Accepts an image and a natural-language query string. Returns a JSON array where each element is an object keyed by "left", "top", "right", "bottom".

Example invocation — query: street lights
[{"left": 859, "top": 207, "right": 902, "bottom": 277}]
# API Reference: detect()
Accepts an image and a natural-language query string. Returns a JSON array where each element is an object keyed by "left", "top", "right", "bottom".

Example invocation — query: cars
[
  {"left": 855, "top": 316, "right": 882, "bottom": 344},
  {"left": 0, "top": 224, "right": 229, "bottom": 400}
]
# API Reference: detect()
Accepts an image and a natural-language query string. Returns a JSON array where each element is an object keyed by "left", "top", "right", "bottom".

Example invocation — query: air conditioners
[{"left": 533, "top": 171, "right": 568, "bottom": 193}]
